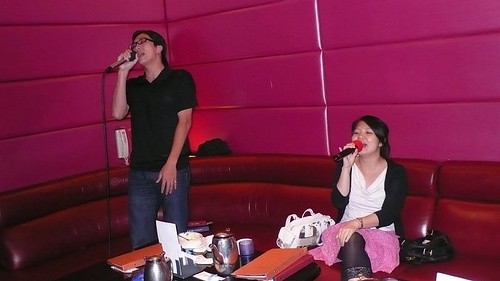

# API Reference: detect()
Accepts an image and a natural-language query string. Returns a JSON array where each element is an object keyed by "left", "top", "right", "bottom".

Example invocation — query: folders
[
  {"left": 232, "top": 247, "right": 308, "bottom": 280},
  {"left": 107, "top": 243, "right": 163, "bottom": 271},
  {"left": 273, "top": 254, "right": 314, "bottom": 281}
]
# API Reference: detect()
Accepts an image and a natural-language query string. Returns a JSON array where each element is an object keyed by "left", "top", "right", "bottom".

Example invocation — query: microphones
[
  {"left": 105, "top": 49, "right": 137, "bottom": 72},
  {"left": 334, "top": 140, "right": 364, "bottom": 162}
]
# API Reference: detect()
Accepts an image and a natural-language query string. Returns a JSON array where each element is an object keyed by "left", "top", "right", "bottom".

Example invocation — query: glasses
[{"left": 128, "top": 37, "right": 157, "bottom": 49}]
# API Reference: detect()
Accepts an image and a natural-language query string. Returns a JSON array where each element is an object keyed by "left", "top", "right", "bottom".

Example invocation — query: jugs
[
  {"left": 209, "top": 232, "right": 239, "bottom": 276},
  {"left": 143, "top": 254, "right": 173, "bottom": 281}
]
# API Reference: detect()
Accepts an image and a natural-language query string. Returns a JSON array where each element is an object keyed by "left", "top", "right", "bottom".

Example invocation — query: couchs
[{"left": 0, "top": 154, "right": 500, "bottom": 281}]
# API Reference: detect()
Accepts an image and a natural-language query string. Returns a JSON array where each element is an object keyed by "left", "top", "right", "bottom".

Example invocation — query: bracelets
[{"left": 356, "top": 218, "right": 363, "bottom": 229}]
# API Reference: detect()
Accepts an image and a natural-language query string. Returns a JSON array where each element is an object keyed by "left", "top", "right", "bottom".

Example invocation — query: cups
[{"left": 239, "top": 240, "right": 255, "bottom": 258}]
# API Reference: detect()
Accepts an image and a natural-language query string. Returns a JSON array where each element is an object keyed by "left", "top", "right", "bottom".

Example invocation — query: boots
[{"left": 344, "top": 265, "right": 373, "bottom": 281}]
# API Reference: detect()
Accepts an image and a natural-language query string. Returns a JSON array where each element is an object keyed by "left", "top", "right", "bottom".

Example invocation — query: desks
[{"left": 57, "top": 251, "right": 320, "bottom": 281}]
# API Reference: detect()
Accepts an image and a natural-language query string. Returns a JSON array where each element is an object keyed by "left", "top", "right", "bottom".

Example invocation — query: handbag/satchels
[
  {"left": 400, "top": 229, "right": 453, "bottom": 266},
  {"left": 195, "top": 138, "right": 231, "bottom": 159},
  {"left": 276, "top": 210, "right": 336, "bottom": 250}
]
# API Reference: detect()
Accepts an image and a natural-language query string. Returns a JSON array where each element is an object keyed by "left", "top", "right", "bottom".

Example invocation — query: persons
[
  {"left": 330, "top": 114, "right": 407, "bottom": 281},
  {"left": 111, "top": 29, "right": 200, "bottom": 249}
]
